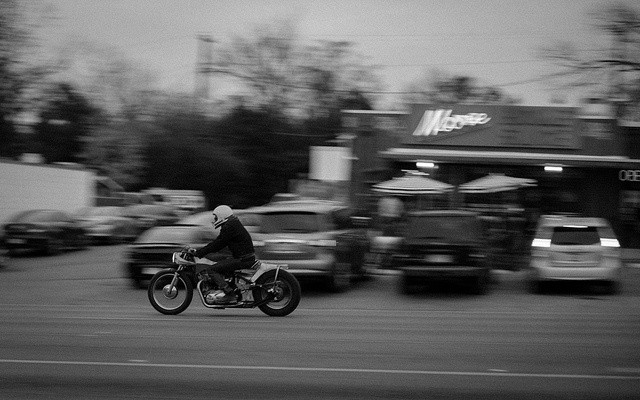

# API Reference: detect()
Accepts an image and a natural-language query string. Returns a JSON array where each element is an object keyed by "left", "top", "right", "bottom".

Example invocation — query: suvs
[
  {"left": 249, "top": 198, "right": 368, "bottom": 288},
  {"left": 530, "top": 215, "right": 620, "bottom": 291},
  {"left": 398, "top": 212, "right": 489, "bottom": 291}
]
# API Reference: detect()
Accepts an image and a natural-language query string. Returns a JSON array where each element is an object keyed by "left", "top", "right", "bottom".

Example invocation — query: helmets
[{"left": 212, "top": 205, "right": 234, "bottom": 229}]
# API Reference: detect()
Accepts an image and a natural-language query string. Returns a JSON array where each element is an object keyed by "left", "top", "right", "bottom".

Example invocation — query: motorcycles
[{"left": 148, "top": 243, "right": 301, "bottom": 317}]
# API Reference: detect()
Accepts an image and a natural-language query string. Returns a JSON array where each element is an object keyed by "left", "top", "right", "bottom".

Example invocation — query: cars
[
  {"left": 126, "top": 224, "right": 223, "bottom": 288},
  {"left": 0, "top": 210, "right": 92, "bottom": 254},
  {"left": 76, "top": 206, "right": 145, "bottom": 243},
  {"left": 130, "top": 205, "right": 181, "bottom": 228}
]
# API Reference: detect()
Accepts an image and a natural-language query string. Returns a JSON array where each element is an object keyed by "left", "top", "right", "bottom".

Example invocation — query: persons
[{"left": 187, "top": 205, "right": 255, "bottom": 305}]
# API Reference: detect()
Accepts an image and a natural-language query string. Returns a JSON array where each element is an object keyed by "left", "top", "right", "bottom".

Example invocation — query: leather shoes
[{"left": 215, "top": 294, "right": 237, "bottom": 303}]
